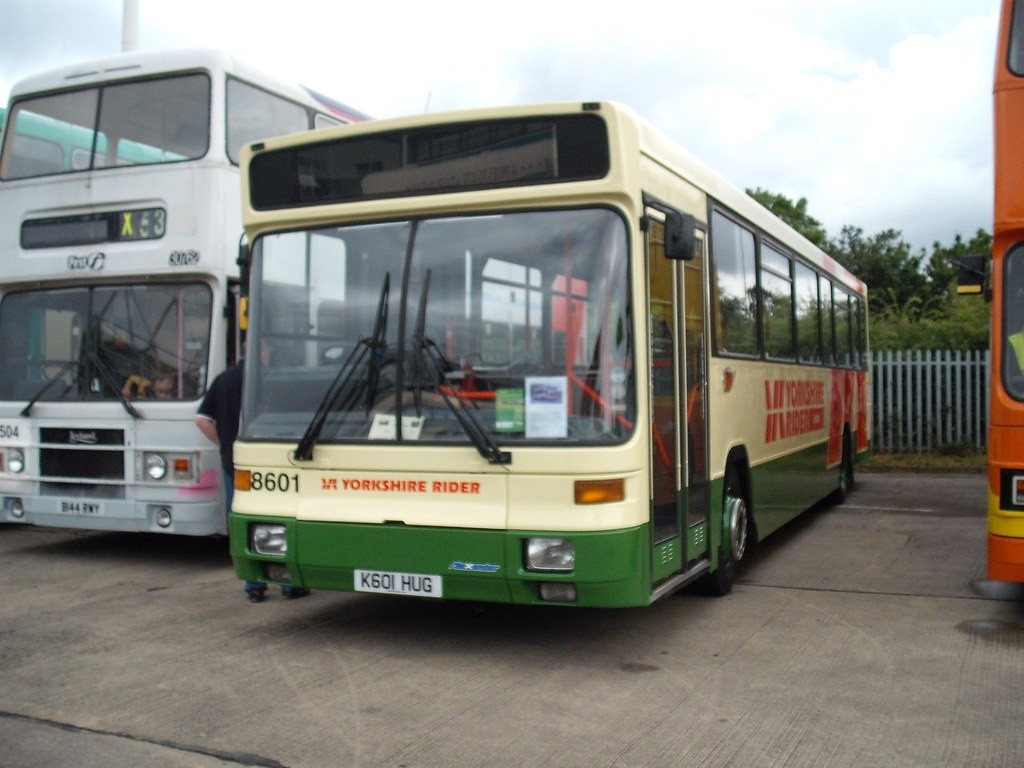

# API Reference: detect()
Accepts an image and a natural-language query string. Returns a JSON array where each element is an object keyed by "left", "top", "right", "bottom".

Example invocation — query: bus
[
  {"left": 227, "top": 98, "right": 879, "bottom": 609},
  {"left": 0, "top": 107, "right": 196, "bottom": 393},
  {"left": 0, "top": 42, "right": 589, "bottom": 539},
  {"left": 949, "top": 1, "right": 1024, "bottom": 602}
]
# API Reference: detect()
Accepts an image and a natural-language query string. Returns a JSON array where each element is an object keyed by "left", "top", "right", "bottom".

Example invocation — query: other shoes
[
  {"left": 282, "top": 587, "right": 311, "bottom": 599},
  {"left": 246, "top": 587, "right": 265, "bottom": 602}
]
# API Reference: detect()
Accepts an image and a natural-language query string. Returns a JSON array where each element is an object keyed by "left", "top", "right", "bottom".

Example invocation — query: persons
[
  {"left": 195, "top": 340, "right": 309, "bottom": 602},
  {"left": 123, "top": 368, "right": 175, "bottom": 399}
]
